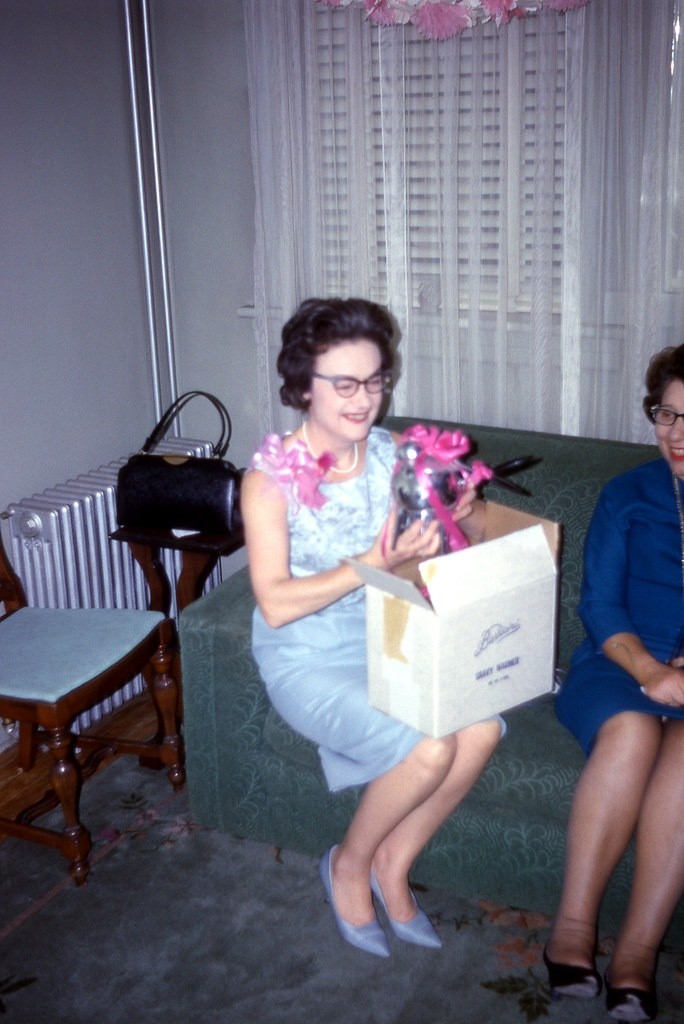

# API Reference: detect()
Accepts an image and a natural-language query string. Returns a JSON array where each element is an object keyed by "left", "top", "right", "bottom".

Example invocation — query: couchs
[{"left": 181, "top": 418, "right": 684, "bottom": 951}]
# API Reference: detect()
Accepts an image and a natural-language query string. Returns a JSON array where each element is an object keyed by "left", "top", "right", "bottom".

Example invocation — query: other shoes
[
  {"left": 543, "top": 942, "right": 602, "bottom": 1000},
  {"left": 603, "top": 968, "right": 658, "bottom": 1024}
]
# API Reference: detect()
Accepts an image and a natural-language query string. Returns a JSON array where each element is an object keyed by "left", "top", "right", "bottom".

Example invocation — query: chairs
[{"left": 0, "top": 531, "right": 183, "bottom": 885}]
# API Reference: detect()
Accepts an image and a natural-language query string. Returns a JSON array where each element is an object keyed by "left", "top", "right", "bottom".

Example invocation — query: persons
[
  {"left": 541, "top": 341, "right": 684, "bottom": 1022},
  {"left": 239, "top": 294, "right": 501, "bottom": 957}
]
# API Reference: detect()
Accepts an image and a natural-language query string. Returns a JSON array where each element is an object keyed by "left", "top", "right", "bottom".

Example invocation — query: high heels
[
  {"left": 319, "top": 845, "right": 391, "bottom": 960},
  {"left": 369, "top": 866, "right": 443, "bottom": 951}
]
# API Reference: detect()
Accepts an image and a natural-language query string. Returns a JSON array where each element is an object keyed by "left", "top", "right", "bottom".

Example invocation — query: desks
[{"left": 111, "top": 527, "right": 245, "bottom": 770}]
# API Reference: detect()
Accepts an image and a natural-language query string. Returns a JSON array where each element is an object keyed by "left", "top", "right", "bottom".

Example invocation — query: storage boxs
[{"left": 345, "top": 498, "right": 560, "bottom": 739}]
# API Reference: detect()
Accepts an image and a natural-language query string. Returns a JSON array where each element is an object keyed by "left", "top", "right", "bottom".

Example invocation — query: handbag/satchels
[{"left": 115, "top": 391, "right": 239, "bottom": 536}]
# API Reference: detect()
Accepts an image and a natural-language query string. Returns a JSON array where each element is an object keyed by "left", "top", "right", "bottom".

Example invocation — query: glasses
[
  {"left": 651, "top": 404, "right": 684, "bottom": 426},
  {"left": 311, "top": 368, "right": 393, "bottom": 398}
]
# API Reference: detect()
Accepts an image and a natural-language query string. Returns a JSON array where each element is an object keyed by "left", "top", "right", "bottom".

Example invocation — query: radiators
[{"left": 1, "top": 439, "right": 221, "bottom": 735}]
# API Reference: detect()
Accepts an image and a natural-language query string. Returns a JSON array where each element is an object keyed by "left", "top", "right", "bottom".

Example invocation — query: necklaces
[
  {"left": 303, "top": 418, "right": 359, "bottom": 474},
  {"left": 671, "top": 470, "right": 684, "bottom": 587}
]
